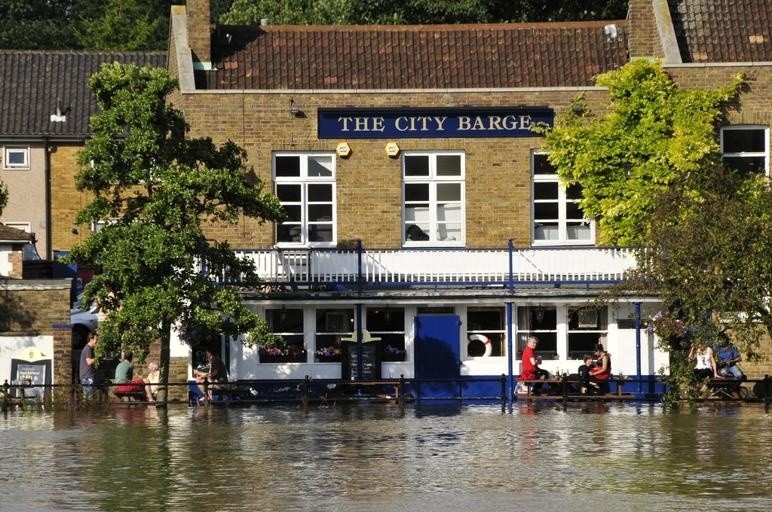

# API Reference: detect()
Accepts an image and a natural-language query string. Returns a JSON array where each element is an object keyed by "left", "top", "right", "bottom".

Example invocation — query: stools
[{"left": 112, "top": 390, "right": 145, "bottom": 409}]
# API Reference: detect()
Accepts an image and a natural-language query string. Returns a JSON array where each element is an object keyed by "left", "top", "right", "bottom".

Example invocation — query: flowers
[{"left": 266, "top": 336, "right": 288, "bottom": 355}]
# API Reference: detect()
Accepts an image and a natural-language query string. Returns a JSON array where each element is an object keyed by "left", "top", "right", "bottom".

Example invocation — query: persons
[
  {"left": 522, "top": 336, "right": 549, "bottom": 396},
  {"left": 578, "top": 344, "right": 611, "bottom": 395},
  {"left": 79, "top": 332, "right": 103, "bottom": 398},
  {"left": 193, "top": 345, "right": 228, "bottom": 402},
  {"left": 115, "top": 350, "right": 161, "bottom": 402},
  {"left": 688, "top": 333, "right": 747, "bottom": 395}
]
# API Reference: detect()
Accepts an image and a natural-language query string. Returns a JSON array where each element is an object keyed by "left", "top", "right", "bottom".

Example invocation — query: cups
[{"left": 537, "top": 356, "right": 542, "bottom": 364}]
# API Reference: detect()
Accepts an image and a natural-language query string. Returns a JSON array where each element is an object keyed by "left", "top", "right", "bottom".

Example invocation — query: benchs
[
  {"left": 318, "top": 378, "right": 410, "bottom": 405},
  {"left": 524, "top": 377, "right": 634, "bottom": 413},
  {"left": 693, "top": 376, "right": 747, "bottom": 399}
]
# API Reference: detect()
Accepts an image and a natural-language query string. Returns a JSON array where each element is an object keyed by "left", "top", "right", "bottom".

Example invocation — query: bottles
[{"left": 556, "top": 366, "right": 559, "bottom": 378}]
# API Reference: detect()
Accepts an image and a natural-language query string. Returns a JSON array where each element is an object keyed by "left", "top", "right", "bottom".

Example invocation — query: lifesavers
[{"left": 467, "top": 334, "right": 492, "bottom": 357}]
